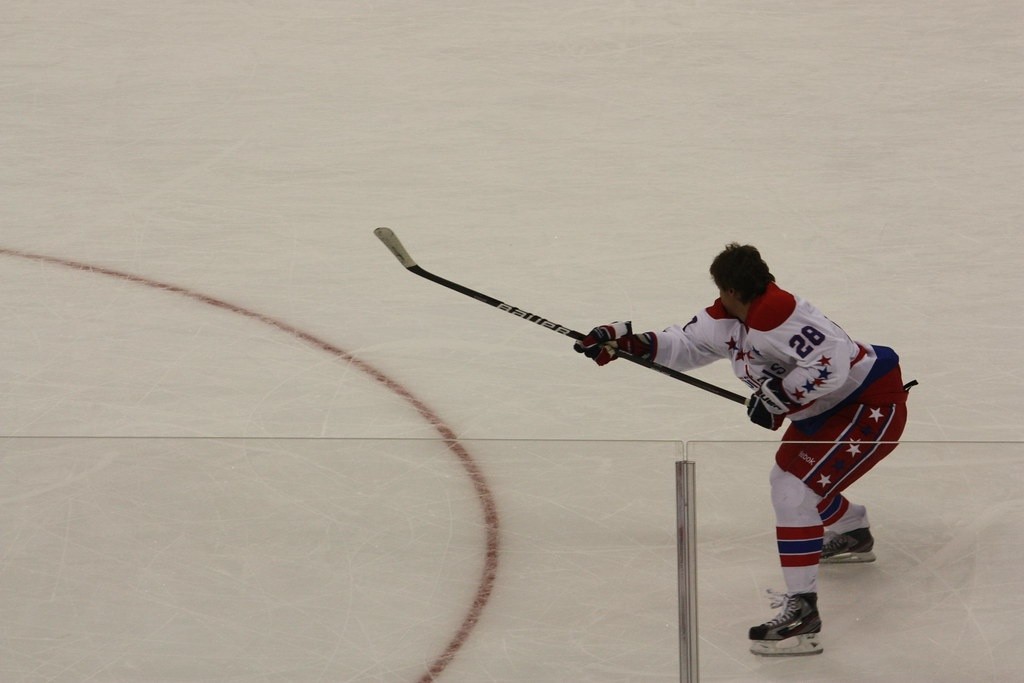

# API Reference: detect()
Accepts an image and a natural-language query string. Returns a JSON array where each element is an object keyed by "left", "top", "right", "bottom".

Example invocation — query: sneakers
[
  {"left": 747, "top": 591, "right": 825, "bottom": 656},
  {"left": 821, "top": 528, "right": 876, "bottom": 563}
]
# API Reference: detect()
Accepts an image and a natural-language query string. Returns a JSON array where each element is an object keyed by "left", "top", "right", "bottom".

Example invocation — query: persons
[{"left": 573, "top": 239, "right": 918, "bottom": 657}]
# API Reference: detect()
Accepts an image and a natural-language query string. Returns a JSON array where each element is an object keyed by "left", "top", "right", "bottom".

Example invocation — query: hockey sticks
[{"left": 373, "top": 226, "right": 751, "bottom": 403}]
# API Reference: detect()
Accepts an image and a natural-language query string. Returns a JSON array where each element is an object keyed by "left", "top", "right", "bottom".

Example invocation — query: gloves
[
  {"left": 747, "top": 379, "right": 796, "bottom": 430},
  {"left": 573, "top": 320, "right": 637, "bottom": 366}
]
